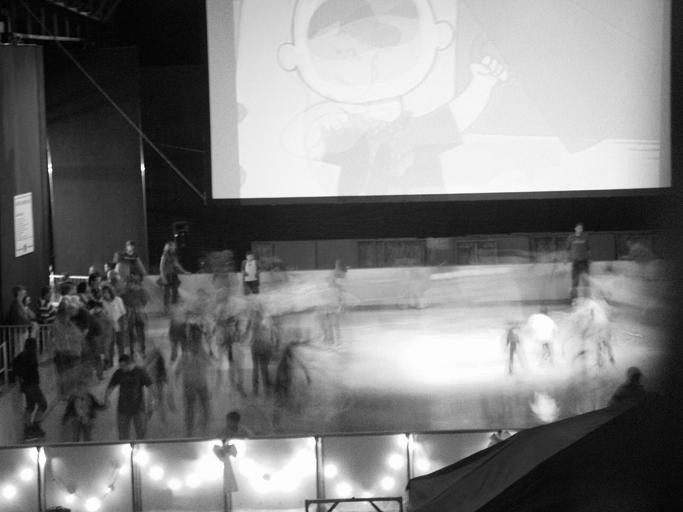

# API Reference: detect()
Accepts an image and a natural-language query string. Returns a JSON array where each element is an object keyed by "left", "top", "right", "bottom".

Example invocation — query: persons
[{"left": 0, "top": 224, "right": 667, "bottom": 444}]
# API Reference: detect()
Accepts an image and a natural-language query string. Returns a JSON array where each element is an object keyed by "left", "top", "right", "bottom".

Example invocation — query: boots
[{"left": 22, "top": 419, "right": 48, "bottom": 440}]
